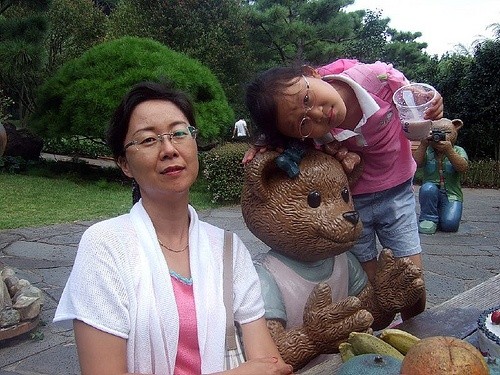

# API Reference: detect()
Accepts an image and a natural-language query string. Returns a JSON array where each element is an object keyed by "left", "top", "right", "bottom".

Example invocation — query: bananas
[{"left": 338, "top": 329, "right": 421, "bottom": 362}]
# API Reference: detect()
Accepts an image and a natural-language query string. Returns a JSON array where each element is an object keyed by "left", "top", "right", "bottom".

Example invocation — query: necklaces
[{"left": 159, "top": 239, "right": 189, "bottom": 253}]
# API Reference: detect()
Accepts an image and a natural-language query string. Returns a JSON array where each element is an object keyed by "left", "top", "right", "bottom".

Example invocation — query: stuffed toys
[
  {"left": 240, "top": 142, "right": 425, "bottom": 369},
  {"left": 413, "top": 117, "right": 469, "bottom": 233}
]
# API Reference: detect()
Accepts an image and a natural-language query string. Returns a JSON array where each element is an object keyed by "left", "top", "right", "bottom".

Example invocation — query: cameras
[{"left": 430, "top": 130, "right": 446, "bottom": 143}]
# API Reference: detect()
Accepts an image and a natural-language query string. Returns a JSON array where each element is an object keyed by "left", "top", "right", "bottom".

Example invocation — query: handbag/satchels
[{"left": 223, "top": 230, "right": 246, "bottom": 370}]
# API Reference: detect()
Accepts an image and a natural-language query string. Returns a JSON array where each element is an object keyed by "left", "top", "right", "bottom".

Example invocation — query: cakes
[{"left": 477, "top": 305, "right": 500, "bottom": 358}]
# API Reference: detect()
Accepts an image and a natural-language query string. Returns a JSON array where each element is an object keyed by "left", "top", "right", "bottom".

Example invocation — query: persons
[
  {"left": 232, "top": 114, "right": 250, "bottom": 141},
  {"left": 242, "top": 58, "right": 443, "bottom": 328},
  {"left": 53, "top": 81, "right": 292, "bottom": 375}
]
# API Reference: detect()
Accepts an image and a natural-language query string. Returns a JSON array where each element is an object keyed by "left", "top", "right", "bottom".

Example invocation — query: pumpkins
[{"left": 401, "top": 336, "right": 489, "bottom": 375}]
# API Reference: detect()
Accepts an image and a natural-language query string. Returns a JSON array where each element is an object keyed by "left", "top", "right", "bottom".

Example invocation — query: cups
[{"left": 392, "top": 82, "right": 436, "bottom": 142}]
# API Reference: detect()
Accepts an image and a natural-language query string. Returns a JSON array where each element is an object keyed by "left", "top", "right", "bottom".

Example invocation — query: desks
[{"left": 293, "top": 274, "right": 500, "bottom": 375}]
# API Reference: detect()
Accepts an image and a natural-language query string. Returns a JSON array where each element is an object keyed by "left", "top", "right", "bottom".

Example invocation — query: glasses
[
  {"left": 121, "top": 126, "right": 198, "bottom": 154},
  {"left": 299, "top": 74, "right": 315, "bottom": 141}
]
